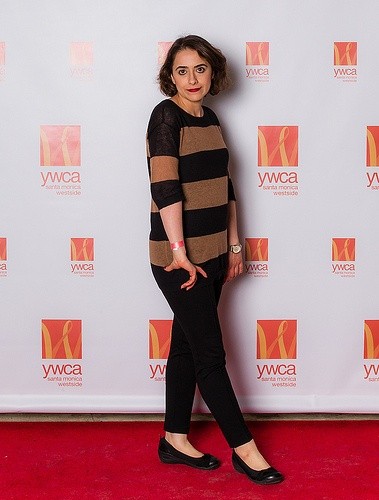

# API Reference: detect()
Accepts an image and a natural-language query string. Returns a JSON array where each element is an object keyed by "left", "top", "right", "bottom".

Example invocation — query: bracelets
[{"left": 170, "top": 240, "right": 184, "bottom": 249}]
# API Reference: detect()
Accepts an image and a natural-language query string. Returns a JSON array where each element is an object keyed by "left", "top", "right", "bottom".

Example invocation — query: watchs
[{"left": 228, "top": 243, "right": 242, "bottom": 254}]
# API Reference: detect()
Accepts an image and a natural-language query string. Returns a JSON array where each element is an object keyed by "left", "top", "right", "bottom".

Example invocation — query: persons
[{"left": 146, "top": 36, "right": 284, "bottom": 485}]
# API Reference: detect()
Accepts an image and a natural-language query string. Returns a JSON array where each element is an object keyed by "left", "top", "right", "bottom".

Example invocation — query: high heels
[
  {"left": 231, "top": 449, "right": 283, "bottom": 484},
  {"left": 158, "top": 437, "right": 220, "bottom": 469}
]
